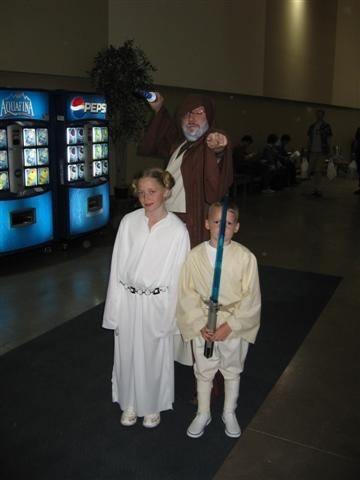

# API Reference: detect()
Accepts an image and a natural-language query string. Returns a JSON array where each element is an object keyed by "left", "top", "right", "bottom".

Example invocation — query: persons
[
  {"left": 102, "top": 168, "right": 191, "bottom": 428},
  {"left": 176, "top": 198, "right": 262, "bottom": 438},
  {"left": 232, "top": 134, "right": 256, "bottom": 173},
  {"left": 353, "top": 126, "right": 360, "bottom": 195},
  {"left": 306, "top": 110, "right": 334, "bottom": 196},
  {"left": 255, "top": 134, "right": 297, "bottom": 192},
  {"left": 135, "top": 92, "right": 234, "bottom": 405}
]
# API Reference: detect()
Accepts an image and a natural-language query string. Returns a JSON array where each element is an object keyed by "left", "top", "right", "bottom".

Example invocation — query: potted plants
[{"left": 88, "top": 38, "right": 161, "bottom": 225}]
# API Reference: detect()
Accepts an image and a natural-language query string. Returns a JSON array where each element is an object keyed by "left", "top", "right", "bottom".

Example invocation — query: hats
[{"left": 172, "top": 95, "right": 214, "bottom": 131}]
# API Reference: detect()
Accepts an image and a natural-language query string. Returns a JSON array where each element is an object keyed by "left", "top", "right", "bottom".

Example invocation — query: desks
[{"left": 325, "top": 159, "right": 350, "bottom": 179}]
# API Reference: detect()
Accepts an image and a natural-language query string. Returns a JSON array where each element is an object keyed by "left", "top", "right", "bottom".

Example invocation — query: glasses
[{"left": 184, "top": 108, "right": 207, "bottom": 118}]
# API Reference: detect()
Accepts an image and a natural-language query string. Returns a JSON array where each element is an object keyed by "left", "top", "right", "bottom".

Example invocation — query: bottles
[{"left": 143, "top": 92, "right": 157, "bottom": 102}]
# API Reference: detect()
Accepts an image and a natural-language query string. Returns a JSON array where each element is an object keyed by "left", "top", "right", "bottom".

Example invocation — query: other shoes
[
  {"left": 187, "top": 411, "right": 211, "bottom": 438},
  {"left": 222, "top": 412, "right": 241, "bottom": 439},
  {"left": 121, "top": 412, "right": 137, "bottom": 426},
  {"left": 312, "top": 191, "right": 321, "bottom": 198},
  {"left": 143, "top": 412, "right": 160, "bottom": 428},
  {"left": 261, "top": 189, "right": 275, "bottom": 193}
]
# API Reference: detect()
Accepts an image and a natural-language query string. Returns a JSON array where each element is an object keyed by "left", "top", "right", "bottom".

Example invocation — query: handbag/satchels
[{"left": 327, "top": 158, "right": 337, "bottom": 181}]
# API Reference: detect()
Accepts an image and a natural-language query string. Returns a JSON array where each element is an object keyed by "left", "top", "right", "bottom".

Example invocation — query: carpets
[{"left": 0, "top": 265, "right": 344, "bottom": 480}]
